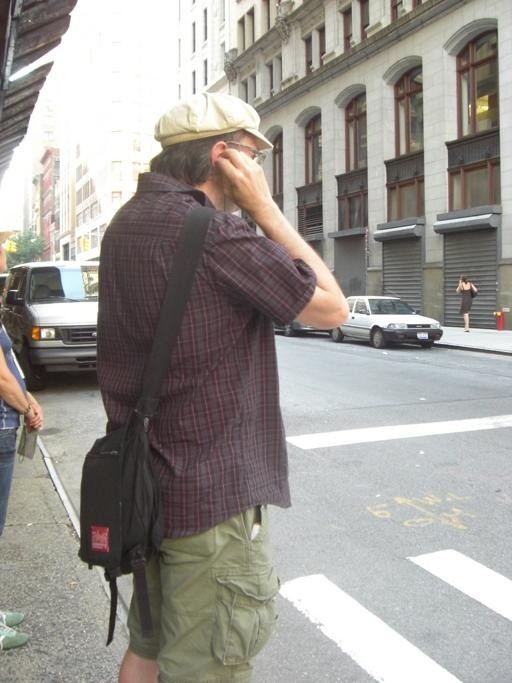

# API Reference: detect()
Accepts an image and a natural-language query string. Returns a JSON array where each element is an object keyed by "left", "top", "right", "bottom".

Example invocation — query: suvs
[{"left": 0, "top": 258, "right": 103, "bottom": 393}]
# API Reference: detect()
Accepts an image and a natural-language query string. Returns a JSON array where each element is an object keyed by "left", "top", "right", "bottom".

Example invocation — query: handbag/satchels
[
  {"left": 469, "top": 282, "right": 478, "bottom": 298},
  {"left": 71, "top": 419, "right": 171, "bottom": 586}
]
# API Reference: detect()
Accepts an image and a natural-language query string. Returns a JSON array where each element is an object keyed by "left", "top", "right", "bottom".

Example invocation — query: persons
[
  {"left": 95, "top": 94, "right": 350, "bottom": 683},
  {"left": 0, "top": 223, "right": 43, "bottom": 652},
  {"left": 456, "top": 274, "right": 478, "bottom": 332}
]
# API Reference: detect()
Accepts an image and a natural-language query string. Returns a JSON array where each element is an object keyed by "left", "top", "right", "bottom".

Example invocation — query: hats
[{"left": 150, "top": 87, "right": 280, "bottom": 154}]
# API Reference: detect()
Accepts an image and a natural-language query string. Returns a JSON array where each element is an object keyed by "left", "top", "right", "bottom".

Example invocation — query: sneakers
[
  {"left": 0, "top": 610, "right": 25, "bottom": 627},
  {"left": 0, "top": 623, "right": 32, "bottom": 650}
]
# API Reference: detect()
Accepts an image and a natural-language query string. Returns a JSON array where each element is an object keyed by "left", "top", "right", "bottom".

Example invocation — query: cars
[
  {"left": 0, "top": 272, "right": 10, "bottom": 307},
  {"left": 272, "top": 318, "right": 323, "bottom": 337},
  {"left": 327, "top": 294, "right": 445, "bottom": 351}
]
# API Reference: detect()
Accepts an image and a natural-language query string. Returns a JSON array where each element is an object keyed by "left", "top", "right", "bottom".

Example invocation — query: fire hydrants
[{"left": 495, "top": 309, "right": 505, "bottom": 329}]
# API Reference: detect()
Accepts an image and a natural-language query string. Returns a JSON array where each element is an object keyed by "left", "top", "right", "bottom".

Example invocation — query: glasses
[{"left": 227, "top": 139, "right": 268, "bottom": 167}]
